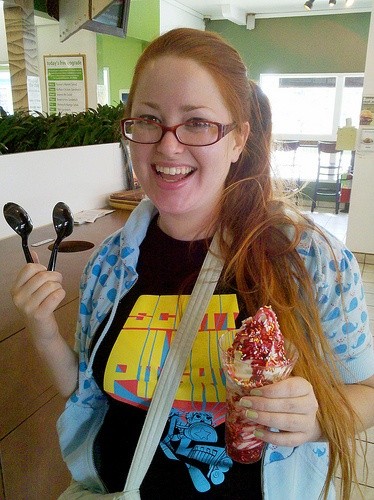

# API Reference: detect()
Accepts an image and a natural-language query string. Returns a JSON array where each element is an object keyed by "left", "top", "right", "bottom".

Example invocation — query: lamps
[
  {"left": 329, "top": 0, "right": 336, "bottom": 7},
  {"left": 304, "top": 0, "right": 315, "bottom": 10}
]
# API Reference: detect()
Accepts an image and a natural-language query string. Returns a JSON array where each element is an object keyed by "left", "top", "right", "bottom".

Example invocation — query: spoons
[
  {"left": 2, "top": 201, "right": 34, "bottom": 263},
  {"left": 46, "top": 201, "right": 74, "bottom": 271}
]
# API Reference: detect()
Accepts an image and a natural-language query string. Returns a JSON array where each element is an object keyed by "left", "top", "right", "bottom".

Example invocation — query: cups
[{"left": 218, "top": 329, "right": 299, "bottom": 463}]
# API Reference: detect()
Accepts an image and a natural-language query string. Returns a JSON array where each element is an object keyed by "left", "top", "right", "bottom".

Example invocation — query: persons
[{"left": 12, "top": 29, "right": 374, "bottom": 500}]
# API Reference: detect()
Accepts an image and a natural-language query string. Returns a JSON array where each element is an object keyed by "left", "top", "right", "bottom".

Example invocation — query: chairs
[
  {"left": 310, "top": 141, "right": 343, "bottom": 215},
  {"left": 269, "top": 140, "right": 301, "bottom": 207}
]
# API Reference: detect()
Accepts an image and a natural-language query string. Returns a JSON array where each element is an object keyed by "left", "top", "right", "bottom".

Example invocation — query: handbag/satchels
[{"left": 56, "top": 478, "right": 141, "bottom": 500}]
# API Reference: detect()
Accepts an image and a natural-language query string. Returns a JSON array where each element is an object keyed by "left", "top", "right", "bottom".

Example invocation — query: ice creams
[{"left": 224, "top": 305, "right": 292, "bottom": 463}]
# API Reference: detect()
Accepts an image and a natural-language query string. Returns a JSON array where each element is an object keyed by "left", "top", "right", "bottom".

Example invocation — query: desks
[
  {"left": 272, "top": 135, "right": 355, "bottom": 213},
  {"left": 0, "top": 207, "right": 135, "bottom": 500}
]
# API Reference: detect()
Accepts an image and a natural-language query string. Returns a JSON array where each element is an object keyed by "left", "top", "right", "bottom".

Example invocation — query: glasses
[{"left": 120, "top": 117, "right": 237, "bottom": 147}]
